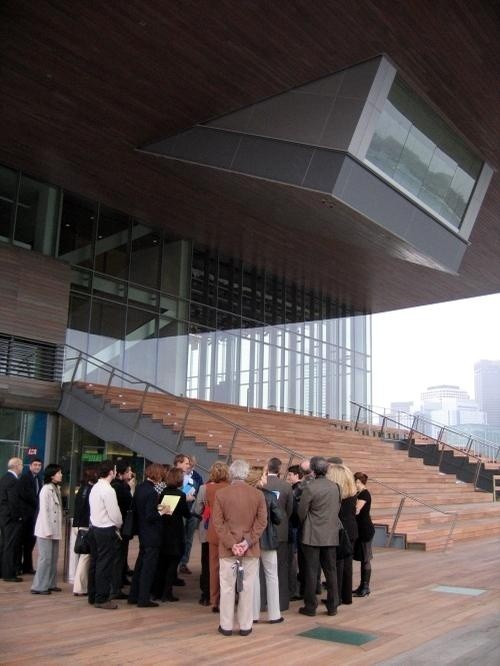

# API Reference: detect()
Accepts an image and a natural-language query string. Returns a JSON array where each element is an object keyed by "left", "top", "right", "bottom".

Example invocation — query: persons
[
  {"left": 72, "top": 456, "right": 204, "bottom": 609},
  {"left": 193, "top": 456, "right": 374, "bottom": 636},
  {"left": 1, "top": 457, "right": 43, "bottom": 582},
  {"left": 31, "top": 464, "right": 62, "bottom": 595}
]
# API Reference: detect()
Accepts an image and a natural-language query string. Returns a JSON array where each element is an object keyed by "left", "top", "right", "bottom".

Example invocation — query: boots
[{"left": 351, "top": 570, "right": 373, "bottom": 598}]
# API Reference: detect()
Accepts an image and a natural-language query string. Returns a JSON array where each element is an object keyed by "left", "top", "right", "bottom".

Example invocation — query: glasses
[{"left": 231, "top": 560, "right": 241, "bottom": 570}]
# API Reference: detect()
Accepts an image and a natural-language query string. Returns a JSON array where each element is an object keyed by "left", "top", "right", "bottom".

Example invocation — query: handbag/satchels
[
  {"left": 73, "top": 528, "right": 92, "bottom": 556},
  {"left": 120, "top": 506, "right": 136, "bottom": 540},
  {"left": 235, "top": 567, "right": 245, "bottom": 594}
]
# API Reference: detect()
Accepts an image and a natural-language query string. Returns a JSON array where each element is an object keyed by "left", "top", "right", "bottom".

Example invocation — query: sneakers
[
  {"left": 251, "top": 617, "right": 285, "bottom": 624},
  {"left": 3, "top": 565, "right": 222, "bottom": 612},
  {"left": 217, "top": 625, "right": 233, "bottom": 636},
  {"left": 239, "top": 627, "right": 253, "bottom": 637},
  {"left": 289, "top": 580, "right": 353, "bottom": 617}
]
